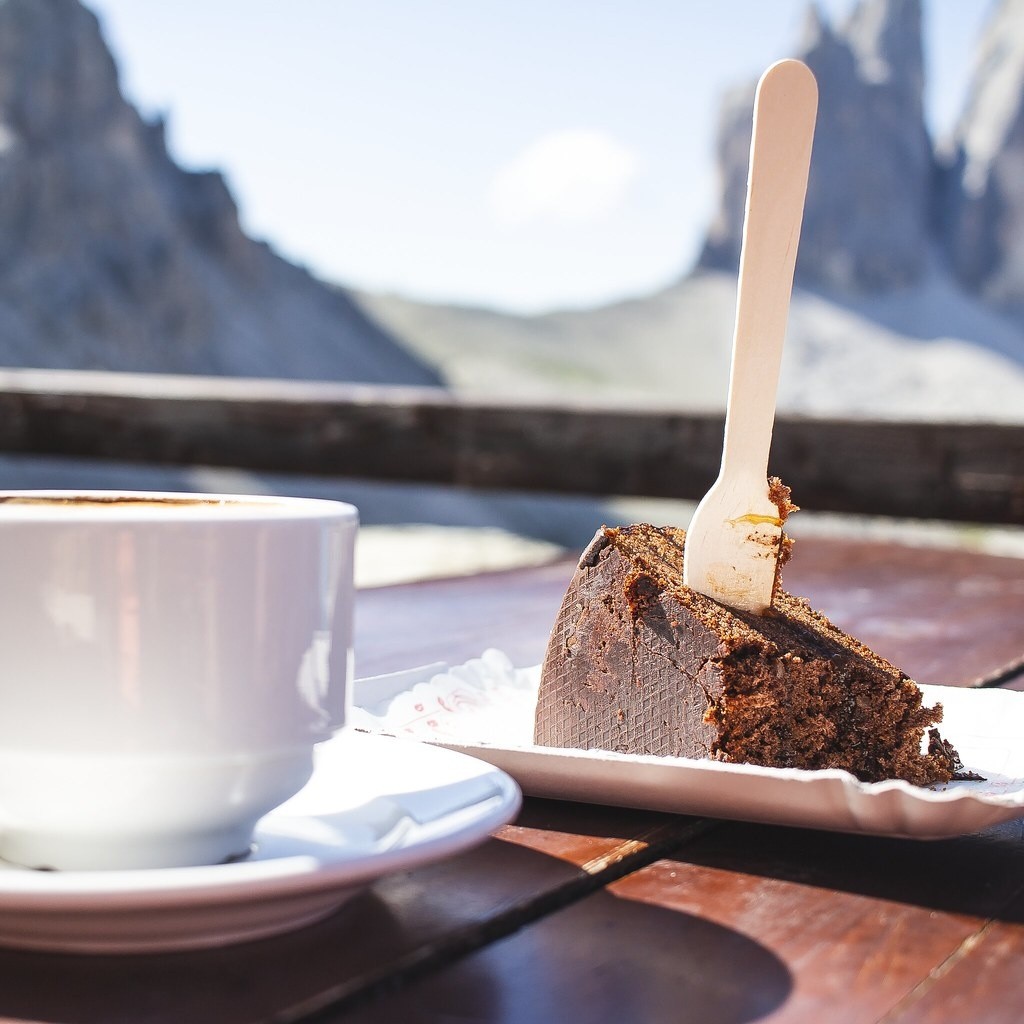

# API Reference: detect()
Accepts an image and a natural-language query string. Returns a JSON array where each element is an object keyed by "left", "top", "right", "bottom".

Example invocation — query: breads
[{"left": 530, "top": 522, "right": 986, "bottom": 786}]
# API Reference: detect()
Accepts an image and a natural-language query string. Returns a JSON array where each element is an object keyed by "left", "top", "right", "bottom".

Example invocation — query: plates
[
  {"left": 0, "top": 732, "right": 524, "bottom": 951},
  {"left": 384, "top": 663, "right": 1024, "bottom": 837}
]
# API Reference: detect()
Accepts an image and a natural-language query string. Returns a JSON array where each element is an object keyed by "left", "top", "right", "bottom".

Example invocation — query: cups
[{"left": 1, "top": 491, "right": 361, "bottom": 874}]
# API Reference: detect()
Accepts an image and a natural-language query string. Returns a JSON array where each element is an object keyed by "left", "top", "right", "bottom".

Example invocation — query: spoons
[{"left": 686, "top": 61, "right": 817, "bottom": 608}]
